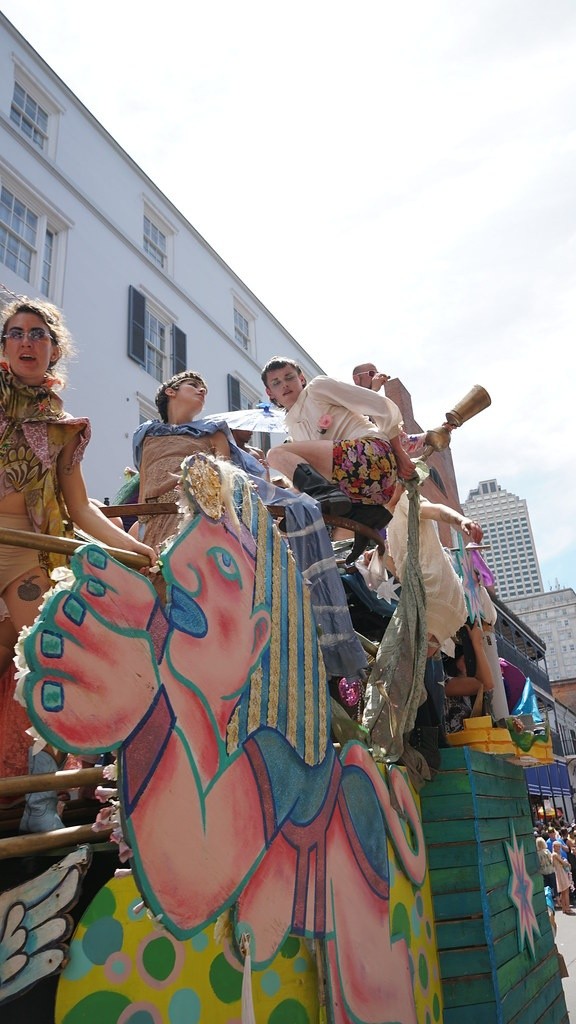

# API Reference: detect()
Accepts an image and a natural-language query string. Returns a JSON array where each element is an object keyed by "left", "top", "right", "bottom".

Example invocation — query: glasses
[
  {"left": 356, "top": 370, "right": 377, "bottom": 376},
  {"left": 3, "top": 327, "right": 52, "bottom": 339}
]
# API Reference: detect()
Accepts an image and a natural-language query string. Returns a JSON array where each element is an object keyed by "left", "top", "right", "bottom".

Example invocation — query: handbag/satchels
[{"left": 564, "top": 859, "right": 570, "bottom": 871}]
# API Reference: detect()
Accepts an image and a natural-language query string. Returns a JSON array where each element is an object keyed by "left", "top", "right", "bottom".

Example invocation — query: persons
[
  {"left": 0, "top": 301, "right": 496, "bottom": 834},
  {"left": 260, "top": 359, "right": 416, "bottom": 533},
  {"left": 533, "top": 814, "right": 576, "bottom": 915}
]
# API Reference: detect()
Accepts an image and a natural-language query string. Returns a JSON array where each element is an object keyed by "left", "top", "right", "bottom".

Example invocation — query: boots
[
  {"left": 343, "top": 504, "right": 393, "bottom": 564},
  {"left": 438, "top": 722, "right": 451, "bottom": 748},
  {"left": 278, "top": 463, "right": 351, "bottom": 532},
  {"left": 402, "top": 725, "right": 441, "bottom": 779},
  {"left": 20, "top": 750, "right": 63, "bottom": 832}
]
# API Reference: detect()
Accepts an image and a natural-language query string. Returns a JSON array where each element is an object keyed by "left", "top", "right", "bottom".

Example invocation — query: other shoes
[{"left": 563, "top": 910, "right": 575, "bottom": 914}]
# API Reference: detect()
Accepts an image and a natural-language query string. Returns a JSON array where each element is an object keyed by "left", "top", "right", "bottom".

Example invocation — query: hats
[
  {"left": 536, "top": 821, "right": 543, "bottom": 826},
  {"left": 571, "top": 823, "right": 576, "bottom": 827}
]
